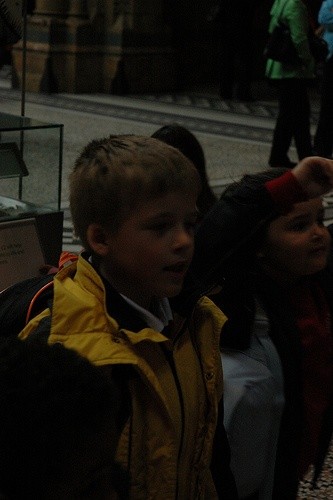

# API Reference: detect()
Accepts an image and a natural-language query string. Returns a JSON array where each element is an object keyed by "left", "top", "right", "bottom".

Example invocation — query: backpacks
[{"left": 1, "top": 250, "right": 129, "bottom": 499}]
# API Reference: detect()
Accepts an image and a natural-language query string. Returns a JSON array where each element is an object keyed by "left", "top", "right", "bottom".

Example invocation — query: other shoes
[{"left": 268, "top": 158, "right": 297, "bottom": 169}]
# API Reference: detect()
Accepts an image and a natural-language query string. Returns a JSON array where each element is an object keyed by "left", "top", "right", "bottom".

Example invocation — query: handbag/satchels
[{"left": 263, "top": 20, "right": 331, "bottom": 79}]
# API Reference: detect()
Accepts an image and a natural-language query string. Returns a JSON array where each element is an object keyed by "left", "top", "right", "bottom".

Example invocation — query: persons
[
  {"left": 152, "top": 122, "right": 218, "bottom": 224},
  {"left": 314, "top": 0, "right": 333, "bottom": 158},
  {"left": 0, "top": 134, "right": 230, "bottom": 500},
  {"left": 264, "top": 0, "right": 314, "bottom": 168},
  {"left": 175, "top": 156, "right": 333, "bottom": 500}
]
